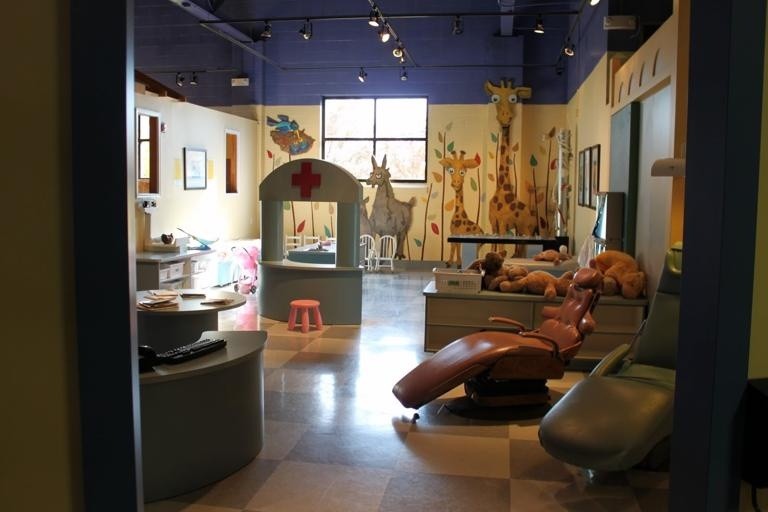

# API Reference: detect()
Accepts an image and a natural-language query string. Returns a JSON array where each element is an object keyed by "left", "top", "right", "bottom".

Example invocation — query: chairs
[
  {"left": 392, "top": 267, "right": 605, "bottom": 409},
  {"left": 538, "top": 241, "right": 683, "bottom": 471},
  {"left": 285, "top": 234, "right": 396, "bottom": 273}
]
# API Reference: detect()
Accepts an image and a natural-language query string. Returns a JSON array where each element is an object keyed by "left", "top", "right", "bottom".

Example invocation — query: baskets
[{"left": 431, "top": 266, "right": 485, "bottom": 295}]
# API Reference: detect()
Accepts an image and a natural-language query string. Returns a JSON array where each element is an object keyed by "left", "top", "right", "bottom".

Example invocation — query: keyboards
[{"left": 156, "top": 338, "right": 226, "bottom": 365}]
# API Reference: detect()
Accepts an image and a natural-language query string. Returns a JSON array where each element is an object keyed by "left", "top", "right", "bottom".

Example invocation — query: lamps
[
  {"left": 564, "top": 44, "right": 576, "bottom": 56},
  {"left": 369, "top": 6, "right": 409, "bottom": 82},
  {"left": 175, "top": 72, "right": 199, "bottom": 87},
  {"left": 534, "top": 23, "right": 545, "bottom": 34},
  {"left": 452, "top": 15, "right": 465, "bottom": 34}
]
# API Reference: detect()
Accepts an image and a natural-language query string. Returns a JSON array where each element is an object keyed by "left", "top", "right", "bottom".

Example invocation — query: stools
[{"left": 288, "top": 299, "right": 323, "bottom": 333}]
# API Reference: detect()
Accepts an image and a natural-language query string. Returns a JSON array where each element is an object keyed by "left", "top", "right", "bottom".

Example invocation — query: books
[{"left": 135, "top": 289, "right": 235, "bottom": 310}]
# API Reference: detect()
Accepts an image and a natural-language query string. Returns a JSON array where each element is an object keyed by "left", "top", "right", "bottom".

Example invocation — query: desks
[
  {"left": 448, "top": 236, "right": 569, "bottom": 267},
  {"left": 423, "top": 280, "right": 648, "bottom": 370},
  {"left": 135, "top": 237, "right": 268, "bottom": 503}
]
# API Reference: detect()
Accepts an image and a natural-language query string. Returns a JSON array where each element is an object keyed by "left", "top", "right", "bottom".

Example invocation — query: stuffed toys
[
  {"left": 596, "top": 250, "right": 644, "bottom": 300},
  {"left": 500, "top": 271, "right": 573, "bottom": 300},
  {"left": 467, "top": 252, "right": 504, "bottom": 275},
  {"left": 488, "top": 266, "right": 528, "bottom": 290},
  {"left": 534, "top": 250, "right": 570, "bottom": 264}
]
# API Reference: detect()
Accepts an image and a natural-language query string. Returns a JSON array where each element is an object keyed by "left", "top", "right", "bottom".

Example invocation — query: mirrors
[
  {"left": 135, "top": 108, "right": 161, "bottom": 199},
  {"left": 224, "top": 129, "right": 240, "bottom": 199}
]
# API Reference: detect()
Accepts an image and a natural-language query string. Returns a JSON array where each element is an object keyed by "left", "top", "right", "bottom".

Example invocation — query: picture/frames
[
  {"left": 184, "top": 147, "right": 207, "bottom": 190},
  {"left": 578, "top": 144, "right": 600, "bottom": 211}
]
